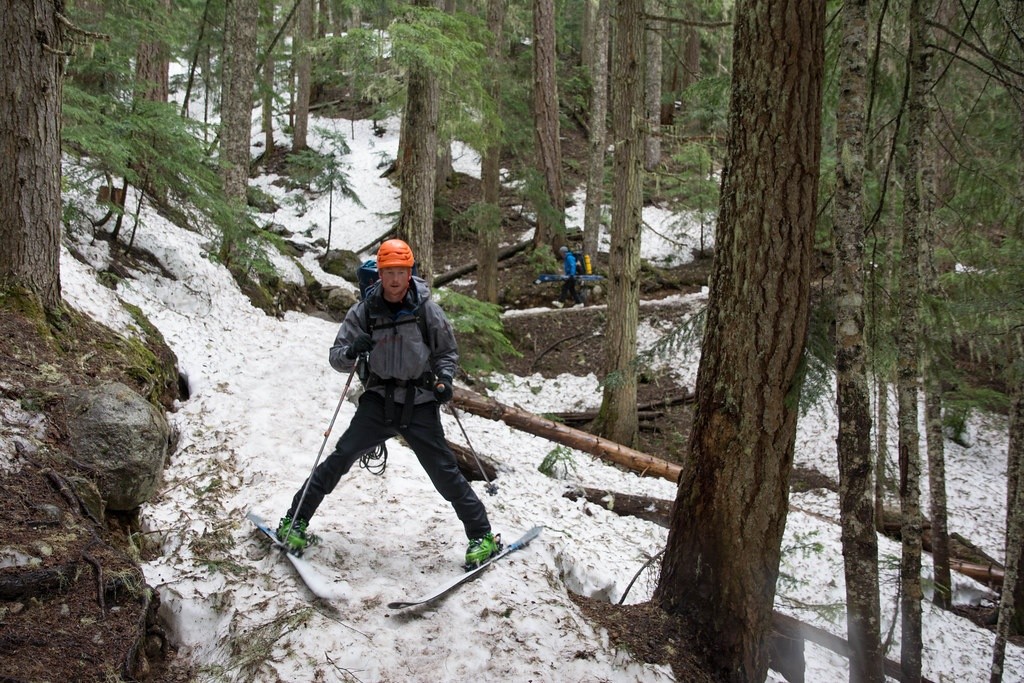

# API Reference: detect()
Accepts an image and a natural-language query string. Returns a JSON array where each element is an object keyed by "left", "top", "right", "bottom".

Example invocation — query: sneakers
[
  {"left": 275, "top": 513, "right": 309, "bottom": 548},
  {"left": 465, "top": 531, "right": 500, "bottom": 564}
]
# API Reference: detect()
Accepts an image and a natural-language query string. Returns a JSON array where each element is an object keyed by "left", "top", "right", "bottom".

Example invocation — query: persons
[
  {"left": 276, "top": 239, "right": 502, "bottom": 565},
  {"left": 552, "top": 247, "right": 584, "bottom": 308}
]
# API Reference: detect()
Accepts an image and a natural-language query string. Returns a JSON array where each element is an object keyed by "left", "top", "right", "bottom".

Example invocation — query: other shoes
[
  {"left": 551, "top": 300, "right": 565, "bottom": 308},
  {"left": 572, "top": 303, "right": 585, "bottom": 309}
]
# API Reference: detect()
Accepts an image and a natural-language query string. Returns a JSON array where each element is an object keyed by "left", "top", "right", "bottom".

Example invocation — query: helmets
[{"left": 377, "top": 238, "right": 415, "bottom": 269}]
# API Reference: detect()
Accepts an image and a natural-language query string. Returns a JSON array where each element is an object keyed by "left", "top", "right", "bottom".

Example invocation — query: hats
[{"left": 559, "top": 246, "right": 569, "bottom": 254}]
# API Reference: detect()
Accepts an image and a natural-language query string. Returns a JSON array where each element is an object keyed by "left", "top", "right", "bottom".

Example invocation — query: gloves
[
  {"left": 346, "top": 335, "right": 376, "bottom": 360},
  {"left": 433, "top": 374, "right": 453, "bottom": 404}
]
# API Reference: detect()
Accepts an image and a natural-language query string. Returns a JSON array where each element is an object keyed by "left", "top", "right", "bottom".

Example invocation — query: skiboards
[
  {"left": 538, "top": 274, "right": 604, "bottom": 281},
  {"left": 247, "top": 513, "right": 544, "bottom": 610}
]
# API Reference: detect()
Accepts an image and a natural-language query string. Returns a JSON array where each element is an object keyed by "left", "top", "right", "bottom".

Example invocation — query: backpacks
[
  {"left": 573, "top": 250, "right": 585, "bottom": 275},
  {"left": 356, "top": 259, "right": 431, "bottom": 348}
]
